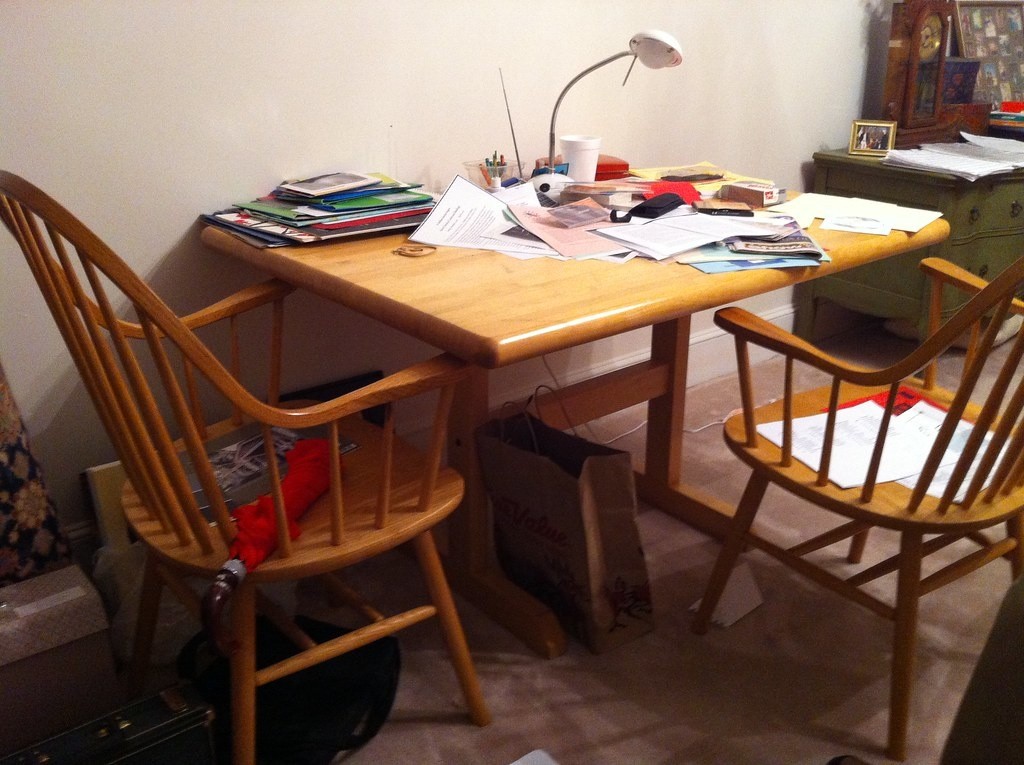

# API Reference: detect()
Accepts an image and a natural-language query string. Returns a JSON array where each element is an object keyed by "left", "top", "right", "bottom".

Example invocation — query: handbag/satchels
[
  {"left": 171, "top": 612, "right": 400, "bottom": 765},
  {"left": 477, "top": 384, "right": 657, "bottom": 655}
]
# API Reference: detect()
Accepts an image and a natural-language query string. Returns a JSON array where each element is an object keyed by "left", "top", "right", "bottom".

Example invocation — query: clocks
[{"left": 886, "top": 0, "right": 964, "bottom": 147}]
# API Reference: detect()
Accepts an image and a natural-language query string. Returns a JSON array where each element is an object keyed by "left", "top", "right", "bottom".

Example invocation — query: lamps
[{"left": 525, "top": 29, "right": 683, "bottom": 204}]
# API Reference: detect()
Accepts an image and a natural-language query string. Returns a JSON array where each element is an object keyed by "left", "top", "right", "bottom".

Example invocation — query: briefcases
[{"left": 0, "top": 687, "right": 218, "bottom": 764}]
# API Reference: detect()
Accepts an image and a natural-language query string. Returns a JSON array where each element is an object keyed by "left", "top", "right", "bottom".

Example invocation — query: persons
[{"left": 857, "top": 127, "right": 888, "bottom": 149}]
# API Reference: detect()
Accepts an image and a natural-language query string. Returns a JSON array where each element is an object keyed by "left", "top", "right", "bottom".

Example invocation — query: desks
[{"left": 200, "top": 160, "right": 953, "bottom": 670}]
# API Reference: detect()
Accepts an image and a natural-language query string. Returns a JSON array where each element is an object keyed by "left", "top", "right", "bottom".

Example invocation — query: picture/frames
[
  {"left": 849, "top": 119, "right": 897, "bottom": 157},
  {"left": 950, "top": 0, "right": 1024, "bottom": 111}
]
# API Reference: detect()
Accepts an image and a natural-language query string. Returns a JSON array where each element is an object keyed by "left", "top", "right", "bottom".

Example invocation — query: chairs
[
  {"left": 692, "top": 257, "right": 1024, "bottom": 762},
  {"left": 0, "top": 169, "right": 491, "bottom": 765}
]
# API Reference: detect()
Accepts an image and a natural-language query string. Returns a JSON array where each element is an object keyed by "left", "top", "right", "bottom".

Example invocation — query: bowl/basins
[{"left": 461, "top": 156, "right": 525, "bottom": 194}]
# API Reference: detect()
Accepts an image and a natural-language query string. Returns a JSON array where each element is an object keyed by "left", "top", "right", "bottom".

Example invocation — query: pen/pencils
[{"left": 464, "top": 154, "right": 526, "bottom": 191}]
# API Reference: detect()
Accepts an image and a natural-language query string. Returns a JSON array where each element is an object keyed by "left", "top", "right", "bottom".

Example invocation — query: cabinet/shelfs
[{"left": 803, "top": 144, "right": 1024, "bottom": 390}]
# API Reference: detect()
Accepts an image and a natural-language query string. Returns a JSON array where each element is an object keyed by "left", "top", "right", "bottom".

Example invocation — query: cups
[{"left": 559, "top": 134, "right": 601, "bottom": 182}]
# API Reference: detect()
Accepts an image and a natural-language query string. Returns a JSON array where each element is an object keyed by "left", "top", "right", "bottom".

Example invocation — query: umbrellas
[{"left": 208, "top": 440, "right": 344, "bottom": 611}]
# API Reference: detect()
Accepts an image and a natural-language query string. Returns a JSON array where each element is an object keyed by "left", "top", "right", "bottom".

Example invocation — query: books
[
  {"left": 560, "top": 185, "right": 644, "bottom": 212},
  {"left": 219, "top": 173, "right": 442, "bottom": 243}
]
